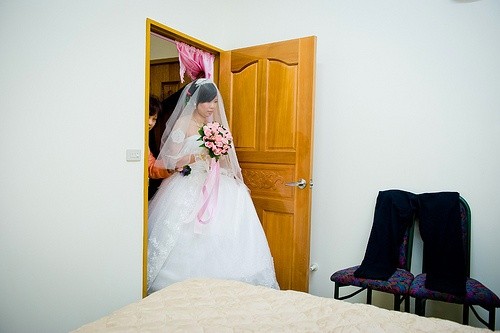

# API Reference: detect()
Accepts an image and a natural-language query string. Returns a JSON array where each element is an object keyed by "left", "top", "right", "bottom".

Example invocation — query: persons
[
  {"left": 147, "top": 79, "right": 281, "bottom": 297},
  {"left": 148, "top": 93, "right": 204, "bottom": 179}
]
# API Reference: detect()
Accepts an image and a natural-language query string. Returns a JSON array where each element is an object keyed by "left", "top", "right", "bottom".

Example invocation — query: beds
[{"left": 70, "top": 280, "right": 494, "bottom": 333}]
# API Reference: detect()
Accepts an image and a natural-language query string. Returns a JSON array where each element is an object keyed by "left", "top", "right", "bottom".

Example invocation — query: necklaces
[{"left": 191, "top": 116, "right": 204, "bottom": 127}]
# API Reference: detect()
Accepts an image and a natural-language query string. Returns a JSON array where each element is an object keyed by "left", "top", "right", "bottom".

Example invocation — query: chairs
[
  {"left": 331, "top": 190, "right": 417, "bottom": 311},
  {"left": 409, "top": 192, "right": 500, "bottom": 330}
]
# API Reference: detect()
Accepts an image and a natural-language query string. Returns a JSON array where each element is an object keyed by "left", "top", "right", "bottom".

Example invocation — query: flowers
[{"left": 196, "top": 122, "right": 233, "bottom": 163}]
